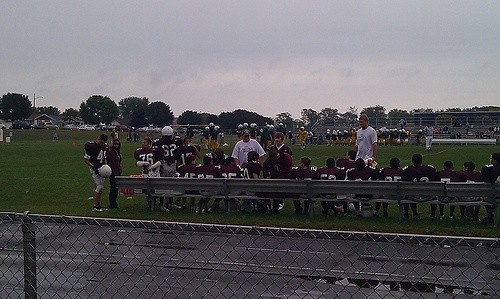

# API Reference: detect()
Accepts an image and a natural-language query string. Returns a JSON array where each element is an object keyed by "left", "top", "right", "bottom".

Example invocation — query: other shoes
[
  {"left": 109, "top": 205, "right": 117, "bottom": 209},
  {"left": 202, "top": 209, "right": 206, "bottom": 214},
  {"left": 302, "top": 146, "right": 306, "bottom": 149},
  {"left": 300, "top": 146, "right": 304, "bottom": 150},
  {"left": 159, "top": 206, "right": 170, "bottom": 212},
  {"left": 103, "top": 207, "right": 109, "bottom": 211},
  {"left": 195, "top": 207, "right": 201, "bottom": 213},
  {"left": 168, "top": 204, "right": 182, "bottom": 210},
  {"left": 278, "top": 203, "right": 285, "bottom": 211},
  {"left": 92, "top": 207, "right": 103, "bottom": 212}
]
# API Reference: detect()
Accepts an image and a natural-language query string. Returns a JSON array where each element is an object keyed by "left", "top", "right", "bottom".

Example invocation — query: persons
[{"left": 53, "top": 113, "right": 500, "bottom": 224}]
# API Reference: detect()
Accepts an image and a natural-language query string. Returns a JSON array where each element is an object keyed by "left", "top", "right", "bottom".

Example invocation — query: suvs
[{"left": 42, "top": 122, "right": 59, "bottom": 130}]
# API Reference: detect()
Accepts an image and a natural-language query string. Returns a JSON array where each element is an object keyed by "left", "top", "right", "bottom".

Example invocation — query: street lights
[{"left": 33, "top": 93, "right": 44, "bottom": 107}]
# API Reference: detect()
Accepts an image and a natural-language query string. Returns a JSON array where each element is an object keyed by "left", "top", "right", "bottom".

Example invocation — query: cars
[
  {"left": 94, "top": 125, "right": 201, "bottom": 134},
  {"left": 79, "top": 125, "right": 95, "bottom": 131},
  {"left": 12, "top": 122, "right": 33, "bottom": 129},
  {"left": 64, "top": 124, "right": 79, "bottom": 131}
]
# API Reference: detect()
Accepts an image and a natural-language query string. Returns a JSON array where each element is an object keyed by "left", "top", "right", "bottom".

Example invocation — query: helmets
[
  {"left": 161, "top": 125, "right": 174, "bottom": 137},
  {"left": 300, "top": 127, "right": 304, "bottom": 131},
  {"left": 95, "top": 164, "right": 112, "bottom": 178},
  {"left": 205, "top": 123, "right": 283, "bottom": 132}
]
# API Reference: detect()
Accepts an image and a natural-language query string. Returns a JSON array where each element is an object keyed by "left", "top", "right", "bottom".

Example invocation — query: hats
[{"left": 241, "top": 129, "right": 250, "bottom": 137}]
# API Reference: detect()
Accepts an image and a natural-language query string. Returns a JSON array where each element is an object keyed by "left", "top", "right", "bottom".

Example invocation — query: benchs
[{"left": 114, "top": 176, "right": 500, "bottom": 223}]
